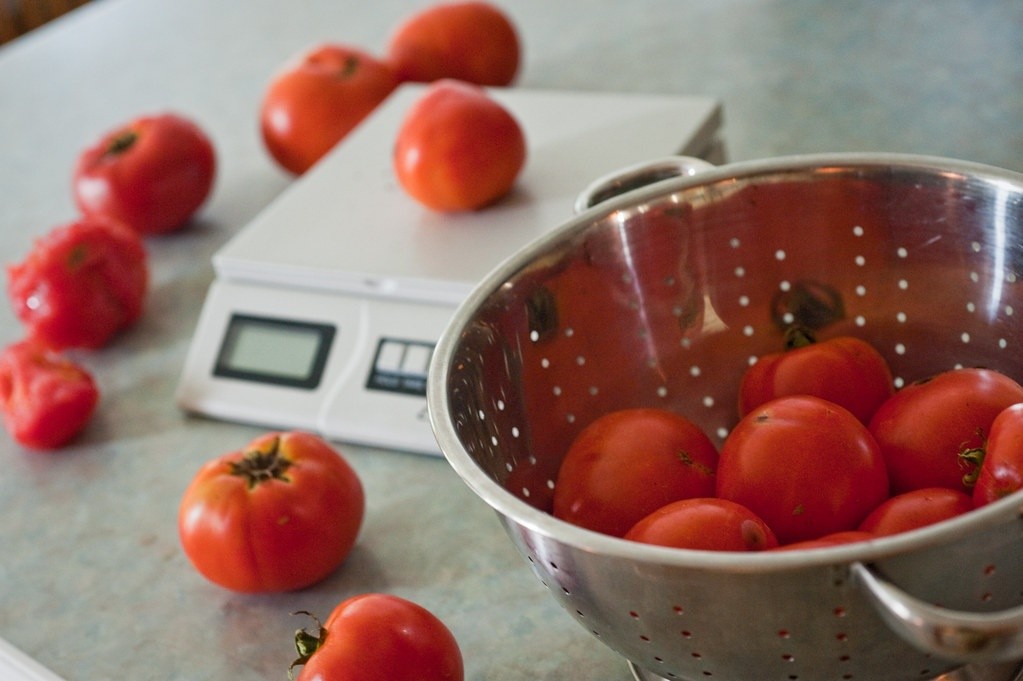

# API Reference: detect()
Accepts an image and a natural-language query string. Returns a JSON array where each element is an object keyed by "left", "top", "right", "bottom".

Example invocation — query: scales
[{"left": 174, "top": 82, "right": 729, "bottom": 460}]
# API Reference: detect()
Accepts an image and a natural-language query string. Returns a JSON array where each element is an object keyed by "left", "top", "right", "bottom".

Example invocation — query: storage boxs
[{"left": 427, "top": 148, "right": 1021, "bottom": 681}]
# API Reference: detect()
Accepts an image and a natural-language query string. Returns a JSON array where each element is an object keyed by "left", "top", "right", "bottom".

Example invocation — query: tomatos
[
  {"left": 0, "top": 1, "right": 525, "bottom": 680},
  {"left": 551, "top": 327, "right": 1023, "bottom": 555}
]
